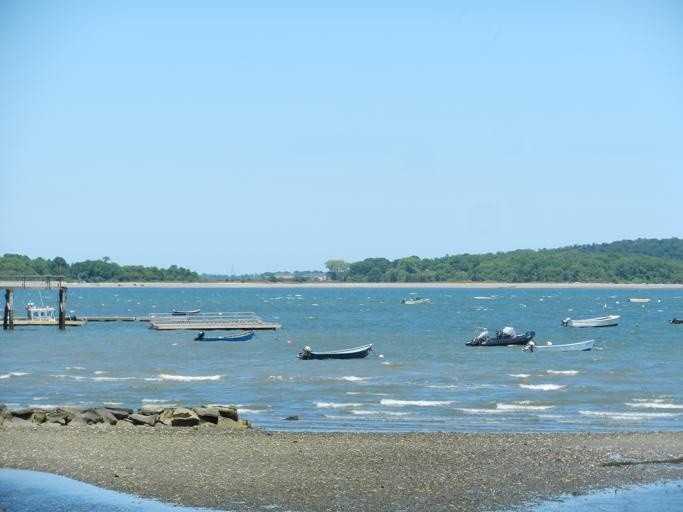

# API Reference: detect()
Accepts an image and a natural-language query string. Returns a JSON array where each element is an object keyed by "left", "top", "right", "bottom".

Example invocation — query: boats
[
  {"left": 400, "top": 297, "right": 429, "bottom": 304},
  {"left": 465, "top": 315, "right": 621, "bottom": 352},
  {"left": 149, "top": 309, "right": 281, "bottom": 342},
  {"left": 296, "top": 344, "right": 373, "bottom": 361},
  {"left": 628, "top": 298, "right": 651, "bottom": 302},
  {"left": 670, "top": 318, "right": 683, "bottom": 324},
  {"left": 0, "top": 302, "right": 87, "bottom": 325}
]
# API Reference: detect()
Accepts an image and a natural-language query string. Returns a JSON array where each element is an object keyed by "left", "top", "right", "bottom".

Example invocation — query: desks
[{"left": 27, "top": 307, "right": 56, "bottom": 320}]
